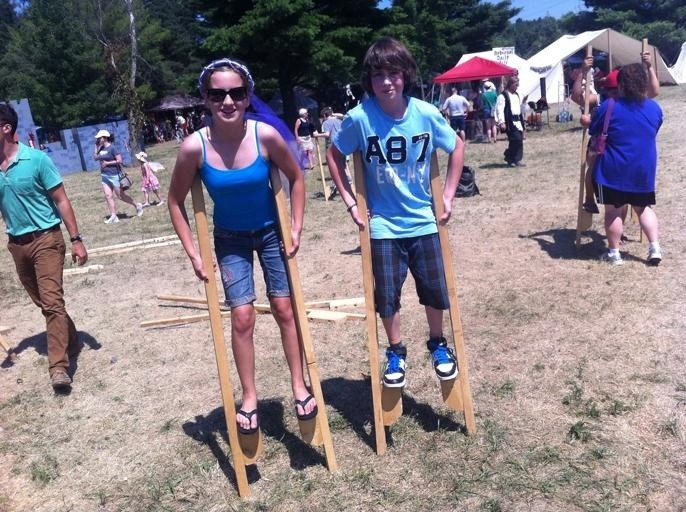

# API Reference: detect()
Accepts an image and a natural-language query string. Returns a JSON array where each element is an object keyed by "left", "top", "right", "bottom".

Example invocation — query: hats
[
  {"left": 94, "top": 130, "right": 110, "bottom": 139},
  {"left": 134, "top": 151, "right": 148, "bottom": 163},
  {"left": 484, "top": 81, "right": 491, "bottom": 88},
  {"left": 605, "top": 69, "right": 621, "bottom": 88}
]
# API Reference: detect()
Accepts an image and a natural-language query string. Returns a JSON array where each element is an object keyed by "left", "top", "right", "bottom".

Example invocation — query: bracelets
[
  {"left": 69, "top": 234, "right": 83, "bottom": 242},
  {"left": 347, "top": 203, "right": 357, "bottom": 212}
]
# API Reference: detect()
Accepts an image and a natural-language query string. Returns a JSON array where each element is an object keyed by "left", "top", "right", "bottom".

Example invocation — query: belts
[{"left": 8, "top": 224, "right": 61, "bottom": 244}]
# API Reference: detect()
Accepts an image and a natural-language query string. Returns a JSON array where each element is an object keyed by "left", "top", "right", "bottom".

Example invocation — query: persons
[
  {"left": 167, "top": 57, "right": 319, "bottom": 433},
  {"left": 570, "top": 53, "right": 660, "bottom": 244},
  {"left": 475, "top": 77, "right": 527, "bottom": 166},
  {"left": 441, "top": 86, "right": 470, "bottom": 144},
  {"left": 1, "top": 105, "right": 87, "bottom": 390},
  {"left": 93, "top": 130, "right": 143, "bottom": 225},
  {"left": 325, "top": 37, "right": 465, "bottom": 391},
  {"left": 135, "top": 151, "right": 165, "bottom": 206},
  {"left": 144, "top": 110, "right": 206, "bottom": 144},
  {"left": 587, "top": 64, "right": 663, "bottom": 264},
  {"left": 295, "top": 108, "right": 353, "bottom": 200},
  {"left": 27, "top": 129, "right": 59, "bottom": 151}
]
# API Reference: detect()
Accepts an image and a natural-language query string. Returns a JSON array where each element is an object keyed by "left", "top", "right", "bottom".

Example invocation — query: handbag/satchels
[
  {"left": 490, "top": 105, "right": 497, "bottom": 117},
  {"left": 119, "top": 172, "right": 133, "bottom": 190}
]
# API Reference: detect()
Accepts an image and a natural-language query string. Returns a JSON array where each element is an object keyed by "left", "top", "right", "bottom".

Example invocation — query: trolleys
[{"left": 556, "top": 84, "right": 573, "bottom": 121}]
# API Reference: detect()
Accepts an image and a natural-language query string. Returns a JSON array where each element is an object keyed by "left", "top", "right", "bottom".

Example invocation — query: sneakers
[
  {"left": 136, "top": 203, "right": 144, "bottom": 217},
  {"left": 598, "top": 252, "right": 624, "bottom": 267},
  {"left": 426, "top": 336, "right": 458, "bottom": 382},
  {"left": 156, "top": 201, "right": 166, "bottom": 207},
  {"left": 104, "top": 214, "right": 120, "bottom": 224},
  {"left": 142, "top": 202, "right": 150, "bottom": 207},
  {"left": 383, "top": 345, "right": 407, "bottom": 389},
  {"left": 50, "top": 370, "right": 72, "bottom": 390},
  {"left": 645, "top": 248, "right": 662, "bottom": 267}
]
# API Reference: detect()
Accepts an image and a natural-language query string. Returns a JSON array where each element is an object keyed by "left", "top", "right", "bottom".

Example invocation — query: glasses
[{"left": 203, "top": 86, "right": 247, "bottom": 102}]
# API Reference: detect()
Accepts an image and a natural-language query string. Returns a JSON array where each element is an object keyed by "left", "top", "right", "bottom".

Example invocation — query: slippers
[
  {"left": 235, "top": 406, "right": 259, "bottom": 435},
  {"left": 293, "top": 394, "right": 318, "bottom": 421}
]
